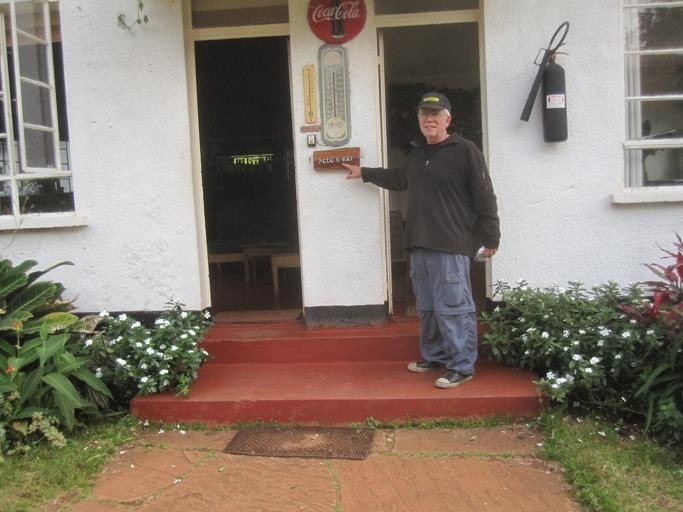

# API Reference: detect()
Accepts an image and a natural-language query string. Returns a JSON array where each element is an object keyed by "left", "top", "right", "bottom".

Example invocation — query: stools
[
  {"left": 209, "top": 242, "right": 247, "bottom": 288},
  {"left": 271, "top": 241, "right": 300, "bottom": 298}
]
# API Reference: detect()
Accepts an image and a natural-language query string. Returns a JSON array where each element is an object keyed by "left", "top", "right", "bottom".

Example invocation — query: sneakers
[
  {"left": 407, "top": 357, "right": 445, "bottom": 374},
  {"left": 434, "top": 367, "right": 476, "bottom": 390}
]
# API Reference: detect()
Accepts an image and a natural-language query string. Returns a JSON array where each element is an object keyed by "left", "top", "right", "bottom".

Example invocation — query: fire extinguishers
[{"left": 519, "top": 21, "right": 570, "bottom": 143}]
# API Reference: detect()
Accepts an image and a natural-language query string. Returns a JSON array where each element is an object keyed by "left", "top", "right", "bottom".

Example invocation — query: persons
[{"left": 340, "top": 90, "right": 500, "bottom": 390}]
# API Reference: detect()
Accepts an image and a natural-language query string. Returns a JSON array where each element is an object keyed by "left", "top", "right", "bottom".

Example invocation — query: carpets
[{"left": 224, "top": 426, "right": 376, "bottom": 459}]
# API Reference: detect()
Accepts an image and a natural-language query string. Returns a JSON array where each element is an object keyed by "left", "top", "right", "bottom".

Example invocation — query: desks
[{"left": 239, "top": 237, "right": 297, "bottom": 283}]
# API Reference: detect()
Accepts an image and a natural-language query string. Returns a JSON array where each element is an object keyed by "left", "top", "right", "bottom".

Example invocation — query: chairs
[{"left": 388, "top": 210, "right": 414, "bottom": 297}]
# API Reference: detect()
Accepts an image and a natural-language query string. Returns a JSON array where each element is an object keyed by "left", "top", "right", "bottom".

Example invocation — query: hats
[{"left": 417, "top": 90, "right": 453, "bottom": 115}]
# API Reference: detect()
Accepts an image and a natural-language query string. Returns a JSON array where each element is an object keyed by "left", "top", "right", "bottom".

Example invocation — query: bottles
[{"left": 329, "top": 0, "right": 347, "bottom": 38}]
